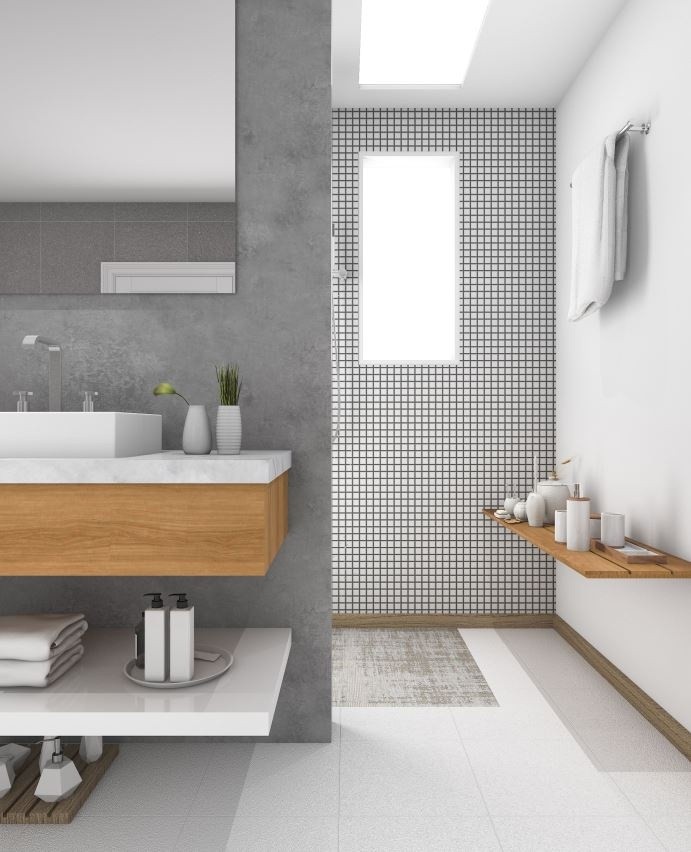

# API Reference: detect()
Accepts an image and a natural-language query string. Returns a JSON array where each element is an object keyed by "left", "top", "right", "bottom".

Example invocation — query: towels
[
  {"left": 565, "top": 129, "right": 631, "bottom": 322},
  {"left": 0, "top": 643, "right": 85, "bottom": 687},
  {"left": 0, "top": 612, "right": 89, "bottom": 661}
]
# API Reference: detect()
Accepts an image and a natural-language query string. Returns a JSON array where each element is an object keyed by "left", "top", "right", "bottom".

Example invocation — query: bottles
[
  {"left": 504, "top": 497, "right": 520, "bottom": 515},
  {"left": 39, "top": 737, "right": 55, "bottom": 776},
  {"left": 514, "top": 502, "right": 528, "bottom": 520},
  {"left": 537, "top": 476, "right": 570, "bottom": 524},
  {"left": 135, "top": 612, "right": 145, "bottom": 668},
  {"left": 79, "top": 736, "right": 104, "bottom": 763},
  {"left": 526, "top": 493, "right": 545, "bottom": 527}
]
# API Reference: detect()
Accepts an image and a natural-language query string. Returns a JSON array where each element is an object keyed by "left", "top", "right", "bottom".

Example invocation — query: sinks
[{"left": 1, "top": 411, "right": 162, "bottom": 458}]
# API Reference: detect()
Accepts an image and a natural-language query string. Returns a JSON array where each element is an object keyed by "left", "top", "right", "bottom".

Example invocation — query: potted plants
[{"left": 215, "top": 362, "right": 245, "bottom": 454}]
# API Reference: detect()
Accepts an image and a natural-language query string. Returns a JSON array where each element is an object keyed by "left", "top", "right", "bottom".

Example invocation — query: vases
[{"left": 182, "top": 405, "right": 214, "bottom": 454}]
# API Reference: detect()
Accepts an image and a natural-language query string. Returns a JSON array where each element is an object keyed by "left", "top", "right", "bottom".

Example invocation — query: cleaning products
[{"left": 133, "top": 612, "right": 145, "bottom": 668}]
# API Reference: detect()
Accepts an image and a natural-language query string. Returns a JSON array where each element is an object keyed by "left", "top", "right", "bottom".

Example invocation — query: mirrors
[{"left": 0, "top": 0, "right": 237, "bottom": 295}]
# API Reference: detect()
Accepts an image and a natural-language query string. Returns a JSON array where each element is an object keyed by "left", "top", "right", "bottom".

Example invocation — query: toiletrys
[
  {"left": 39, "top": 736, "right": 60, "bottom": 776},
  {"left": 168, "top": 593, "right": 194, "bottom": 683},
  {"left": 143, "top": 591, "right": 170, "bottom": 682},
  {"left": 80, "top": 736, "right": 103, "bottom": 763},
  {"left": 33, "top": 736, "right": 82, "bottom": 802}
]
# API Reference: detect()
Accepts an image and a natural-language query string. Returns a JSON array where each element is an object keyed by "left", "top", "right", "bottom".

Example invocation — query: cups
[
  {"left": 589, "top": 513, "right": 601, "bottom": 539},
  {"left": 601, "top": 512, "right": 626, "bottom": 548},
  {"left": 554, "top": 510, "right": 567, "bottom": 544},
  {"left": 566, "top": 496, "right": 592, "bottom": 551}
]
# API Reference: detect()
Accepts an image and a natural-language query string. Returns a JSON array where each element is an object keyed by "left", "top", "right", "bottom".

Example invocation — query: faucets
[{"left": 22, "top": 334, "right": 63, "bottom": 411}]
